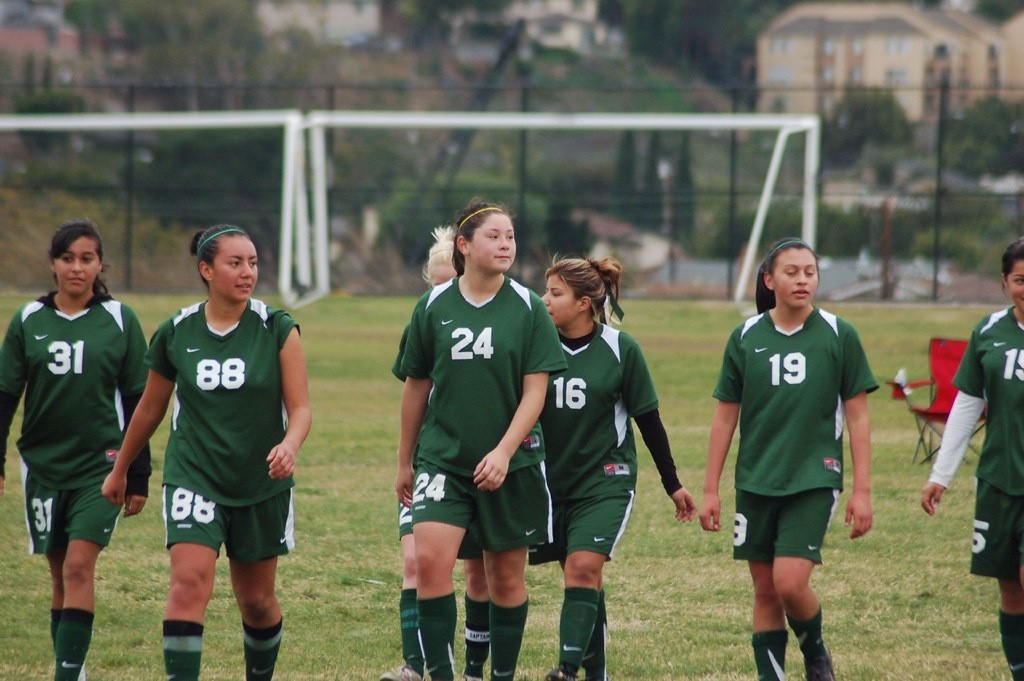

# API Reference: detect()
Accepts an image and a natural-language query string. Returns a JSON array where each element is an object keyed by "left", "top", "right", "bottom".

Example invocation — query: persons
[
  {"left": 921, "top": 239, "right": 1024, "bottom": 681},
  {"left": 100, "top": 221, "right": 314, "bottom": 680},
  {"left": 0, "top": 221, "right": 151, "bottom": 681},
  {"left": 698, "top": 238, "right": 881, "bottom": 681},
  {"left": 378, "top": 199, "right": 697, "bottom": 681}
]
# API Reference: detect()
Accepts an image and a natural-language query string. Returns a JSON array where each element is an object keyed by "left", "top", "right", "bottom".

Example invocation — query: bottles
[{"left": 894, "top": 370, "right": 905, "bottom": 387}]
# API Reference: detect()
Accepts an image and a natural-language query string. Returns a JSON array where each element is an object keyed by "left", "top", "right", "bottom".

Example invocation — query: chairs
[{"left": 886, "top": 335, "right": 991, "bottom": 465}]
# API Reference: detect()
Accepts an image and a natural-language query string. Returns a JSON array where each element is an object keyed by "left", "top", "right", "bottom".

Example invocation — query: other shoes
[
  {"left": 380, "top": 666, "right": 422, "bottom": 681},
  {"left": 802, "top": 647, "right": 835, "bottom": 681},
  {"left": 545, "top": 669, "right": 574, "bottom": 681}
]
